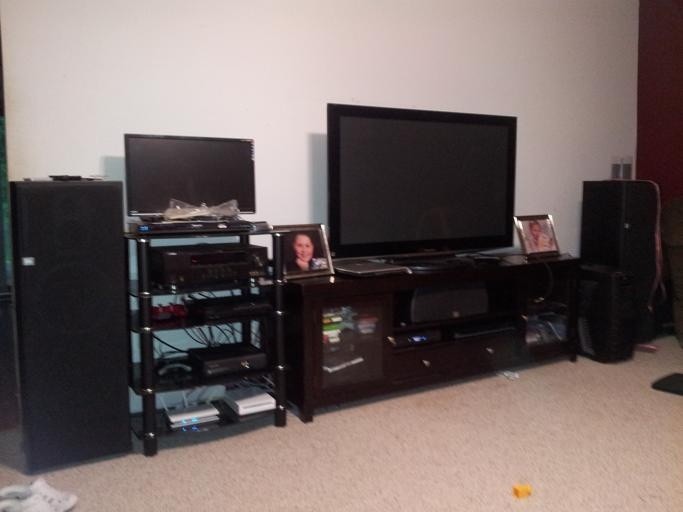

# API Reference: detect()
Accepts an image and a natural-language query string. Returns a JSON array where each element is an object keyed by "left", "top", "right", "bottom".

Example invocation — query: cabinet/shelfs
[
  {"left": 259, "top": 251, "right": 581, "bottom": 424},
  {"left": 124, "top": 229, "right": 290, "bottom": 457}
]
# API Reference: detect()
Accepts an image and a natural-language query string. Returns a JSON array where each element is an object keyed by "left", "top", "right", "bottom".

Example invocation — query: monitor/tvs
[
  {"left": 326, "top": 101, "right": 517, "bottom": 270},
  {"left": 124, "top": 132, "right": 256, "bottom": 216}
]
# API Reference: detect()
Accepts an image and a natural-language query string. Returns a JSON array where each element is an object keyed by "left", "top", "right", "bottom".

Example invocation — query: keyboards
[{"left": 150, "top": 223, "right": 253, "bottom": 234}]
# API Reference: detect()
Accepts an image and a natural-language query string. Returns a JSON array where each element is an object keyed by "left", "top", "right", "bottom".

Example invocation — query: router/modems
[
  {"left": 160, "top": 390, "right": 221, "bottom": 423},
  {"left": 222, "top": 386, "right": 277, "bottom": 416}
]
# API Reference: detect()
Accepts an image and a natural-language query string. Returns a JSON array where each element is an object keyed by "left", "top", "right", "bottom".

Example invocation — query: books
[{"left": 320, "top": 307, "right": 377, "bottom": 374}]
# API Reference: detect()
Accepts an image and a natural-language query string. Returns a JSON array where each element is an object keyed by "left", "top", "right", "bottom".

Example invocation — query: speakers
[
  {"left": 9, "top": 178, "right": 135, "bottom": 473},
  {"left": 581, "top": 180, "right": 663, "bottom": 339}
]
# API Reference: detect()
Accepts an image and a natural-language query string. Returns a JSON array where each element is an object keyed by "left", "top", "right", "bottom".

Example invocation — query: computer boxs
[{"left": 574, "top": 263, "right": 636, "bottom": 365}]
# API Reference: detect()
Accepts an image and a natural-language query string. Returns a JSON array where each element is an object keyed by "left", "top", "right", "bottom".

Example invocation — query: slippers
[{"left": 0, "top": 477, "right": 77, "bottom": 512}]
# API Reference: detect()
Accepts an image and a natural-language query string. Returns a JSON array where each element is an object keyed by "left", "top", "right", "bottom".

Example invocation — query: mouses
[{"left": 252, "top": 221, "right": 269, "bottom": 233}]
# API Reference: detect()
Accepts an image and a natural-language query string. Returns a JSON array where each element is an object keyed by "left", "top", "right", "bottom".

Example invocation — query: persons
[
  {"left": 527, "top": 221, "right": 553, "bottom": 251},
  {"left": 286, "top": 234, "right": 327, "bottom": 273}
]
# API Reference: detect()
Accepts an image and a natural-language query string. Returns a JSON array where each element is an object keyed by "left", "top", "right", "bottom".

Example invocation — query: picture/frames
[
  {"left": 513, "top": 215, "right": 562, "bottom": 259},
  {"left": 272, "top": 223, "right": 335, "bottom": 279}
]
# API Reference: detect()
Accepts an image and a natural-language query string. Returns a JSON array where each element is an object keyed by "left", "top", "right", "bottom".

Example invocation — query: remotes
[{"left": 473, "top": 257, "right": 502, "bottom": 264}]
